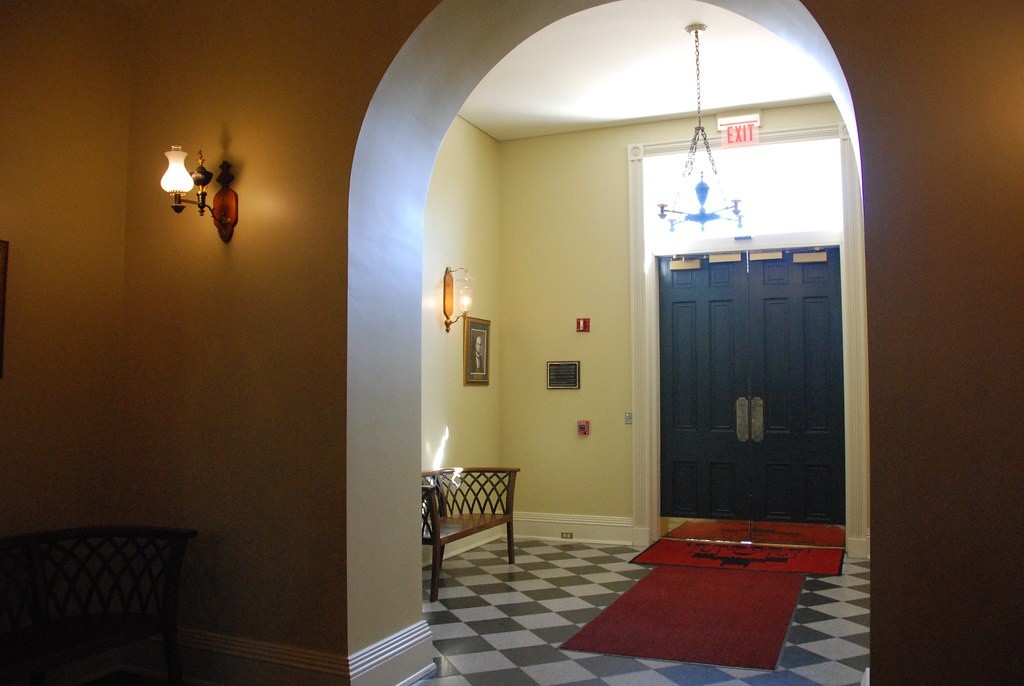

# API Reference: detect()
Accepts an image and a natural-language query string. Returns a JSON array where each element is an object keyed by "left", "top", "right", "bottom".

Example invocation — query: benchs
[
  {"left": 421, "top": 466, "right": 521, "bottom": 601},
  {"left": 0, "top": 525, "right": 198, "bottom": 686}
]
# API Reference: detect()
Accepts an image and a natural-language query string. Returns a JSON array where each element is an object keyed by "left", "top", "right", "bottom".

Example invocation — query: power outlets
[{"left": 560, "top": 531, "right": 573, "bottom": 539}]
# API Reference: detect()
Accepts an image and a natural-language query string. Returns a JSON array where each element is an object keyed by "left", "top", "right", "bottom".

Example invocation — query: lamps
[
  {"left": 656, "top": 22, "right": 748, "bottom": 230},
  {"left": 444, "top": 266, "right": 476, "bottom": 330},
  {"left": 159, "top": 144, "right": 239, "bottom": 242}
]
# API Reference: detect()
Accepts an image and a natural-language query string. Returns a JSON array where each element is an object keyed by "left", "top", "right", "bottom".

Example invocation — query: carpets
[
  {"left": 553, "top": 538, "right": 846, "bottom": 672},
  {"left": 663, "top": 520, "right": 845, "bottom": 545}
]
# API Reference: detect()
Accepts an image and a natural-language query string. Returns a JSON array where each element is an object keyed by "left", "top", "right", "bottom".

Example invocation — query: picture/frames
[{"left": 462, "top": 316, "right": 491, "bottom": 386}]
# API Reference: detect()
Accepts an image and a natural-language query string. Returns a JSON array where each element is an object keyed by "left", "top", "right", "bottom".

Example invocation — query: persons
[{"left": 470, "top": 335, "right": 484, "bottom": 373}]
